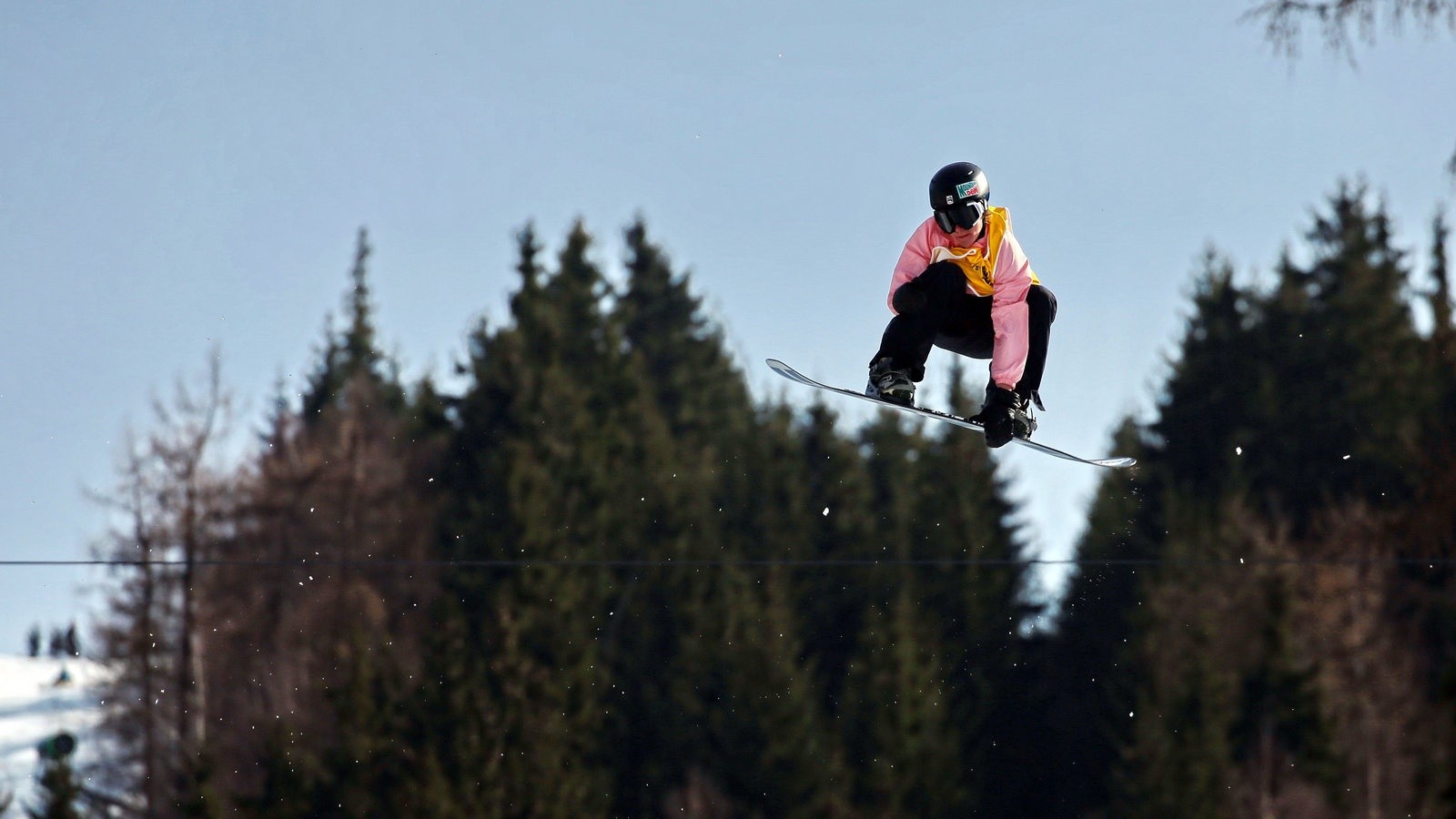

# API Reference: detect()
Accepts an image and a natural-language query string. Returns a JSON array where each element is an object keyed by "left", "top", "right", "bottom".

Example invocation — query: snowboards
[{"left": 765, "top": 357, "right": 1139, "bottom": 469}]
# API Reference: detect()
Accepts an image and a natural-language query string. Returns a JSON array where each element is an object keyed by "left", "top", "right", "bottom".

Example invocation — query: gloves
[
  {"left": 983, "top": 385, "right": 1014, "bottom": 447},
  {"left": 893, "top": 262, "right": 932, "bottom": 316}
]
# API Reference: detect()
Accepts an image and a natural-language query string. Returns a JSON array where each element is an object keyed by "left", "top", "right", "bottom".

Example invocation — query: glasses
[{"left": 934, "top": 201, "right": 984, "bottom": 235}]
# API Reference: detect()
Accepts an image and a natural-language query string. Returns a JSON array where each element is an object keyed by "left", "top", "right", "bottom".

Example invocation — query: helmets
[{"left": 929, "top": 162, "right": 990, "bottom": 209}]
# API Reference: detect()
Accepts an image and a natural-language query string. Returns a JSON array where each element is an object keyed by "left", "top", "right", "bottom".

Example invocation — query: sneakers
[
  {"left": 1011, "top": 392, "right": 1032, "bottom": 437},
  {"left": 865, "top": 364, "right": 914, "bottom": 402}
]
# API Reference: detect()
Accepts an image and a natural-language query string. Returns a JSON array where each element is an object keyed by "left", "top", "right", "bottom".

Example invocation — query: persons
[
  {"left": 30, "top": 626, "right": 39, "bottom": 656},
  {"left": 865, "top": 162, "right": 1057, "bottom": 447}
]
